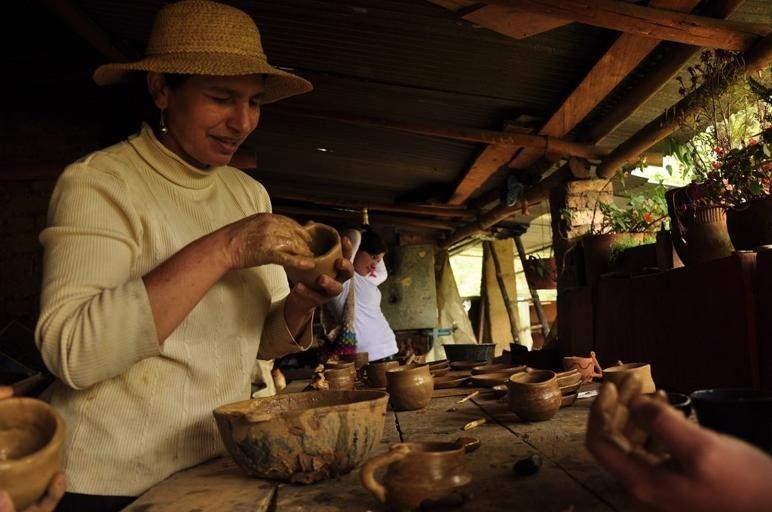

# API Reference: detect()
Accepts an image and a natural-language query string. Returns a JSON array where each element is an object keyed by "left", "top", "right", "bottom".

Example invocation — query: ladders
[{"left": 489, "top": 237, "right": 549, "bottom": 346}]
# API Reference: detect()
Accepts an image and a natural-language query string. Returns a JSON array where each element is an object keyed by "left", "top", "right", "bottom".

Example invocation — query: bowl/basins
[{"left": 213, "top": 388, "right": 390, "bottom": 487}]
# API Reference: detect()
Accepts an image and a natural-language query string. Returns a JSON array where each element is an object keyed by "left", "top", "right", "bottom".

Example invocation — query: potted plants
[
  {"left": 558, "top": 134, "right": 736, "bottom": 286},
  {"left": 523, "top": 253, "right": 557, "bottom": 290}
]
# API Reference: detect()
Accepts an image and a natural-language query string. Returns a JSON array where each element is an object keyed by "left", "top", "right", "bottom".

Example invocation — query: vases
[{"left": 726, "top": 195, "right": 772, "bottom": 251}]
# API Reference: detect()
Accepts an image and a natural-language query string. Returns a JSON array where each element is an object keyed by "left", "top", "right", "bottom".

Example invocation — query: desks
[{"left": 121, "top": 363, "right": 653, "bottom": 512}]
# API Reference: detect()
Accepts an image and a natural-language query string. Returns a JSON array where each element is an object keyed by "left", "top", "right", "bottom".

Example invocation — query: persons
[
  {"left": 1, "top": 472, "right": 67, "bottom": 512},
  {"left": 584, "top": 372, "right": 771, "bottom": 512},
  {"left": 36, "top": 3, "right": 356, "bottom": 510},
  {"left": 326, "top": 232, "right": 400, "bottom": 367}
]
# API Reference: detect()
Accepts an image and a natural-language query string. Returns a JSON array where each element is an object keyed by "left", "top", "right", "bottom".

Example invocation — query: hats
[{"left": 87, "top": 1, "right": 315, "bottom": 107}]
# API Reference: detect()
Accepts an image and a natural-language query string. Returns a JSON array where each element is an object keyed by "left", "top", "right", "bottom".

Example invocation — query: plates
[
  {"left": 418, "top": 359, "right": 527, "bottom": 389},
  {"left": 556, "top": 368, "right": 582, "bottom": 407}
]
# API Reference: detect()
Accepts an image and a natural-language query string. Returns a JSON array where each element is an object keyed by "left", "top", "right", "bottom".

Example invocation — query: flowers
[{"left": 711, "top": 138, "right": 772, "bottom": 203}]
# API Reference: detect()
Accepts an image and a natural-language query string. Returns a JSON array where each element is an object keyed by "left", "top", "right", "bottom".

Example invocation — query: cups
[
  {"left": 356, "top": 360, "right": 400, "bottom": 390},
  {"left": 283, "top": 220, "right": 347, "bottom": 292},
  {"left": 360, "top": 440, "right": 475, "bottom": 512},
  {"left": 563, "top": 356, "right": 594, "bottom": 385},
  {"left": 384, "top": 361, "right": 435, "bottom": 411},
  {"left": 599, "top": 360, "right": 658, "bottom": 398},
  {"left": 507, "top": 369, "right": 562, "bottom": 422}
]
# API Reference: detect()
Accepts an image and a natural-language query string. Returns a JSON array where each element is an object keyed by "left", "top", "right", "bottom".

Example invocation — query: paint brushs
[{"left": 456, "top": 391, "right": 479, "bottom": 404}]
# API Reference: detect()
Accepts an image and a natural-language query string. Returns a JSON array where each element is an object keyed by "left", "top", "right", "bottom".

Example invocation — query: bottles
[{"left": 657, "top": 222, "right": 673, "bottom": 271}]
